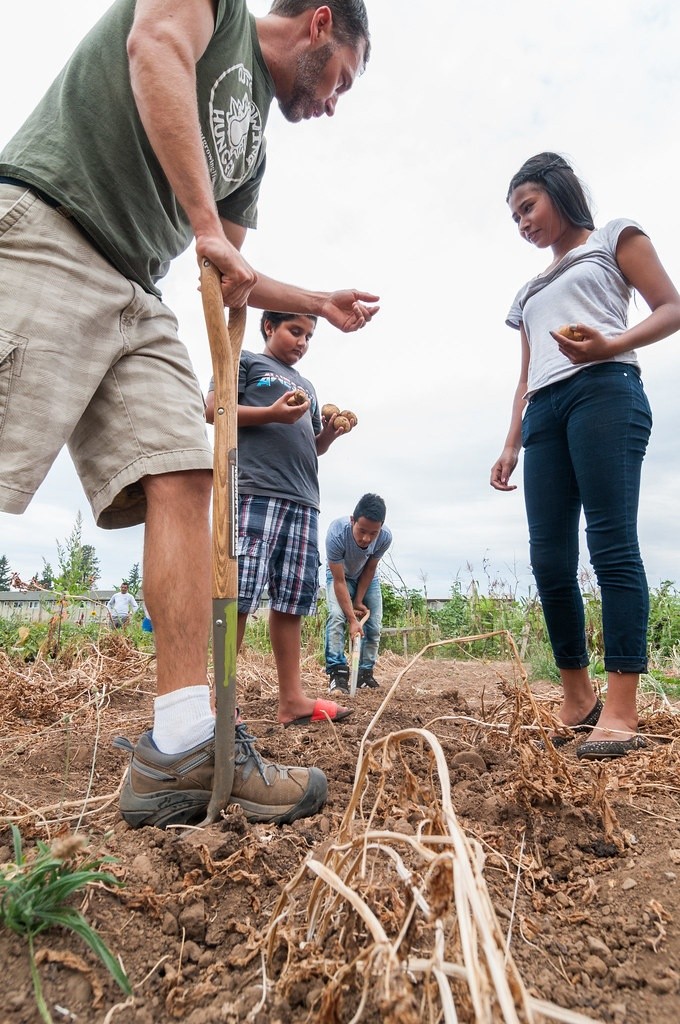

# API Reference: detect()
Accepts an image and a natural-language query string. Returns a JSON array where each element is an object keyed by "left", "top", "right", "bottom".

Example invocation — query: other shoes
[
  {"left": 577, "top": 735, "right": 644, "bottom": 761},
  {"left": 537, "top": 697, "right": 604, "bottom": 751}
]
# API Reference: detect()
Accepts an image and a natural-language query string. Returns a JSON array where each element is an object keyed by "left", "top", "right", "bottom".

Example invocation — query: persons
[
  {"left": 324, "top": 493, "right": 393, "bottom": 693},
  {"left": 203, "top": 307, "right": 355, "bottom": 727},
  {"left": 106, "top": 581, "right": 138, "bottom": 628},
  {"left": 0, "top": 0, "right": 381, "bottom": 827},
  {"left": 489, "top": 150, "right": 680, "bottom": 759}
]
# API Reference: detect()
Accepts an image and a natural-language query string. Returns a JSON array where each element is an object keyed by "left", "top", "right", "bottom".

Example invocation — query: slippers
[{"left": 284, "top": 697, "right": 354, "bottom": 729}]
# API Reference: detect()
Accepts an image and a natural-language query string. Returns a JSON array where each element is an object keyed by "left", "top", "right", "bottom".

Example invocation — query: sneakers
[
  {"left": 329, "top": 665, "right": 350, "bottom": 695},
  {"left": 112, "top": 706, "right": 327, "bottom": 831},
  {"left": 357, "top": 669, "right": 379, "bottom": 688}
]
